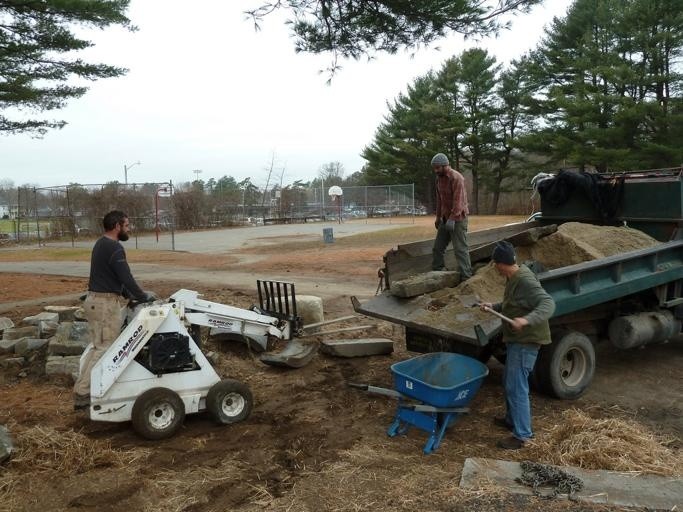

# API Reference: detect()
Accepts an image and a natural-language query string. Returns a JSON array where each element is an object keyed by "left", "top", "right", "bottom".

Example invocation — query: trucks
[{"left": 349, "top": 167, "right": 682, "bottom": 400}]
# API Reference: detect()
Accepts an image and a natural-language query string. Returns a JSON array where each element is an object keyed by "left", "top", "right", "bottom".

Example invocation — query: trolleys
[{"left": 347, "top": 352, "right": 490, "bottom": 454}]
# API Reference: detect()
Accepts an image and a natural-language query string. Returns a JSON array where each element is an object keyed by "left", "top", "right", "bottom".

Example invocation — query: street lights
[
  {"left": 123, "top": 162, "right": 139, "bottom": 191},
  {"left": 192, "top": 169, "right": 201, "bottom": 192}
]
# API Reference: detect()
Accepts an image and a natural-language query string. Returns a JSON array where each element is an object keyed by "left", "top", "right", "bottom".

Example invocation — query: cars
[{"left": 327, "top": 204, "right": 427, "bottom": 221}]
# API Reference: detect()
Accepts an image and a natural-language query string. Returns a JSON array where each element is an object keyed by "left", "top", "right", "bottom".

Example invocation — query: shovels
[{"left": 462, "top": 295, "right": 513, "bottom": 325}]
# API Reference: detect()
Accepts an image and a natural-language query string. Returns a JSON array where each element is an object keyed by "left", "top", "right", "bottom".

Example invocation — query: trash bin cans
[{"left": 323, "top": 228, "right": 333, "bottom": 243}]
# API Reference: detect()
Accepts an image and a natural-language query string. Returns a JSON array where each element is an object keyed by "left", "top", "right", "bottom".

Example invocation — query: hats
[
  {"left": 491, "top": 240, "right": 517, "bottom": 265},
  {"left": 431, "top": 153, "right": 450, "bottom": 166}
]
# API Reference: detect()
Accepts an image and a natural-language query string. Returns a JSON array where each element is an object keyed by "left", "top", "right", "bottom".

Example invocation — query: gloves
[
  {"left": 139, "top": 290, "right": 159, "bottom": 303},
  {"left": 445, "top": 218, "right": 455, "bottom": 234}
]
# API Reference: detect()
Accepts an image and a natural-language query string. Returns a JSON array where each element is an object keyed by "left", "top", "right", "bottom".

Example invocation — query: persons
[
  {"left": 70, "top": 211, "right": 157, "bottom": 407},
  {"left": 430, "top": 153, "right": 472, "bottom": 283},
  {"left": 479, "top": 241, "right": 556, "bottom": 449}
]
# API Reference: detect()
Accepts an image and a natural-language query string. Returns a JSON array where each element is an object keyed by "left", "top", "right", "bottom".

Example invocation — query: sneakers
[
  {"left": 494, "top": 415, "right": 515, "bottom": 428},
  {"left": 72, "top": 391, "right": 90, "bottom": 411},
  {"left": 497, "top": 432, "right": 535, "bottom": 449}
]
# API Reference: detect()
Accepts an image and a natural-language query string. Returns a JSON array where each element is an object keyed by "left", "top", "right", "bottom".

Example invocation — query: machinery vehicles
[{"left": 77, "top": 279, "right": 304, "bottom": 440}]
